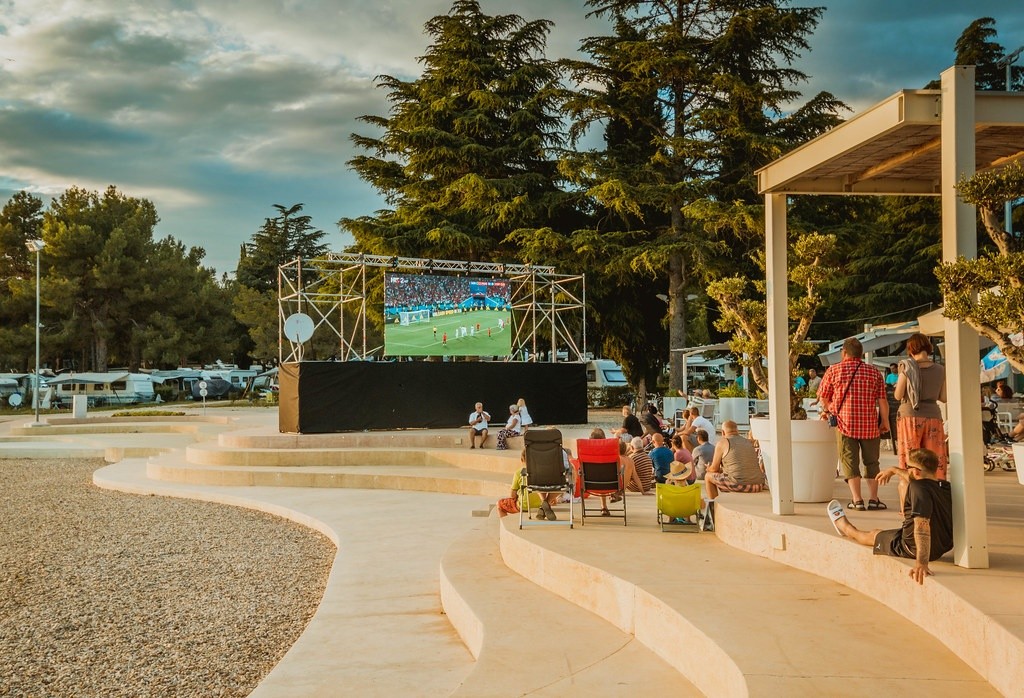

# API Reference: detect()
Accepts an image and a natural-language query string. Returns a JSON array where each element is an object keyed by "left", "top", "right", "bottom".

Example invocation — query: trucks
[
  {"left": 665, "top": 356, "right": 732, "bottom": 384},
  {"left": 0, "top": 364, "right": 279, "bottom": 408},
  {"left": 547, "top": 350, "right": 629, "bottom": 388}
]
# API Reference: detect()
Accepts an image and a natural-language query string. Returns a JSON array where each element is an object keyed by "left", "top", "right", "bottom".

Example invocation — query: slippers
[{"left": 827, "top": 500, "right": 847, "bottom": 536}]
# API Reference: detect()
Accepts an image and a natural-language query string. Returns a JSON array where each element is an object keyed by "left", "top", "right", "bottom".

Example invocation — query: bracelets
[{"left": 823, "top": 410, "right": 829, "bottom": 413}]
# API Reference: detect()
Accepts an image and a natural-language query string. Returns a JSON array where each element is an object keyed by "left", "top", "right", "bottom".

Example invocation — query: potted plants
[
  {"left": 663, "top": 388, "right": 687, "bottom": 419},
  {"left": 934, "top": 159, "right": 1024, "bottom": 486},
  {"left": 706, "top": 232, "right": 840, "bottom": 503},
  {"left": 716, "top": 383, "right": 749, "bottom": 425}
]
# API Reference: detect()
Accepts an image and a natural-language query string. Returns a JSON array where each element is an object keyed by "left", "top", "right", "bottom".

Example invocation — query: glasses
[
  {"left": 840, "top": 348, "right": 844, "bottom": 351},
  {"left": 905, "top": 463, "right": 922, "bottom": 471}
]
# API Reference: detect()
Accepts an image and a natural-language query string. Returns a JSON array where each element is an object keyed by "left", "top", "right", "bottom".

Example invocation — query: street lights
[
  {"left": 230, "top": 353, "right": 234, "bottom": 367},
  {"left": 25, "top": 238, "right": 47, "bottom": 422}
]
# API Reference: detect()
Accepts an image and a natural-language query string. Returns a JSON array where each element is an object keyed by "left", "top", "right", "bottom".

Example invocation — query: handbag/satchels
[{"left": 828, "top": 414, "right": 837, "bottom": 427}]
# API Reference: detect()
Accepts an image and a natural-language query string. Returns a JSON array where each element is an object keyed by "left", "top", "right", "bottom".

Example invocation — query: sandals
[
  {"left": 847, "top": 499, "right": 866, "bottom": 511},
  {"left": 867, "top": 497, "right": 888, "bottom": 509}
]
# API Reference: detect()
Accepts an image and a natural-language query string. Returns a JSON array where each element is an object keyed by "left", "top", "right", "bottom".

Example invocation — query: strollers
[{"left": 981, "top": 399, "right": 1017, "bottom": 472}]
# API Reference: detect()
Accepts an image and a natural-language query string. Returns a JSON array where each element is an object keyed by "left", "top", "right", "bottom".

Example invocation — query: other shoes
[
  {"left": 601, "top": 510, "right": 610, "bottom": 516},
  {"left": 480, "top": 443, "right": 483, "bottom": 448},
  {"left": 536, "top": 508, "right": 545, "bottom": 520},
  {"left": 609, "top": 494, "right": 622, "bottom": 502},
  {"left": 542, "top": 500, "right": 556, "bottom": 521},
  {"left": 471, "top": 446, "right": 475, "bottom": 448}
]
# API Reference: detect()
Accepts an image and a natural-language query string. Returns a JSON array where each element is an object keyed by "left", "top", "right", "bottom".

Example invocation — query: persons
[
  {"left": 663, "top": 460, "right": 692, "bottom": 523},
  {"left": 537, "top": 449, "right": 569, "bottom": 520},
  {"left": 887, "top": 362, "right": 898, "bottom": 385},
  {"left": 497, "top": 448, "right": 545, "bottom": 519},
  {"left": 469, "top": 402, "right": 491, "bottom": 449},
  {"left": 794, "top": 371, "right": 806, "bottom": 390},
  {"left": 827, "top": 448, "right": 954, "bottom": 586},
  {"left": 517, "top": 399, "right": 533, "bottom": 432},
  {"left": 704, "top": 420, "right": 765, "bottom": 500},
  {"left": 808, "top": 369, "right": 821, "bottom": 393},
  {"left": 750, "top": 413, "right": 765, "bottom": 448},
  {"left": 894, "top": 334, "right": 947, "bottom": 480},
  {"left": 678, "top": 389, "right": 710, "bottom": 401},
  {"left": 385, "top": 273, "right": 511, "bottom": 306},
  {"left": 810, "top": 337, "right": 890, "bottom": 511},
  {"left": 562, "top": 405, "right": 716, "bottom": 501},
  {"left": 496, "top": 405, "right": 520, "bottom": 450},
  {"left": 735, "top": 369, "right": 750, "bottom": 389},
  {"left": 1003, "top": 412, "right": 1024, "bottom": 444},
  {"left": 433, "top": 316, "right": 510, "bottom": 343},
  {"left": 590, "top": 428, "right": 622, "bottom": 515},
  {"left": 981, "top": 380, "right": 1013, "bottom": 404}
]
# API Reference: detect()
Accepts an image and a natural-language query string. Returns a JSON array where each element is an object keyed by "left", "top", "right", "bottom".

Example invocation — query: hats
[{"left": 663, "top": 461, "right": 693, "bottom": 480}]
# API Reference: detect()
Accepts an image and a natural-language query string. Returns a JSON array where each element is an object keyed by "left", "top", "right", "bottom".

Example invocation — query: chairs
[
  {"left": 520, "top": 429, "right": 574, "bottom": 529},
  {"left": 575, "top": 436, "right": 627, "bottom": 526},
  {"left": 656, "top": 483, "right": 701, "bottom": 533}
]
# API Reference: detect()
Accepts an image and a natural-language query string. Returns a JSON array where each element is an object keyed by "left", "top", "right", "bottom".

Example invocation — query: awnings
[{"left": 686, "top": 358, "right": 737, "bottom": 372}]
